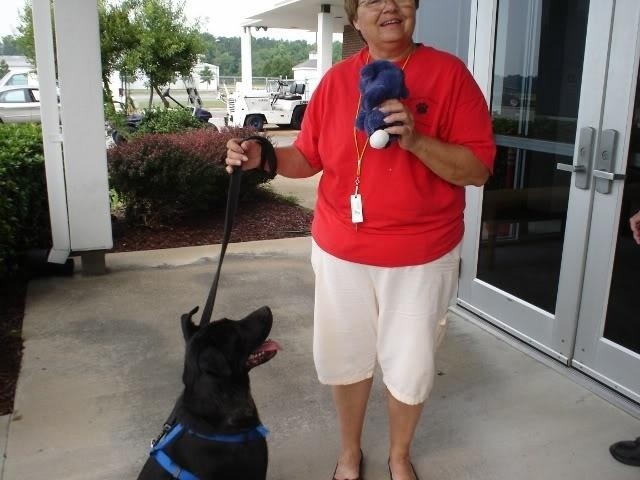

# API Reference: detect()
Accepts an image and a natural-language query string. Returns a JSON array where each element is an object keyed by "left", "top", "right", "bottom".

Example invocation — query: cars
[{"left": 0, "top": 87, "right": 61, "bottom": 125}]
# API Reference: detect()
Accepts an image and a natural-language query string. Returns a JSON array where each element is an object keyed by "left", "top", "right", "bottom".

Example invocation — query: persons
[
  {"left": 610, "top": 209, "right": 640, "bottom": 468},
  {"left": 224, "top": 0, "right": 500, "bottom": 479}
]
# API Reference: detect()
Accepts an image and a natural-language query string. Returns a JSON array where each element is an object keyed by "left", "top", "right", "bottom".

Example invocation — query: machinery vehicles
[{"left": 224, "top": 80, "right": 308, "bottom": 132}]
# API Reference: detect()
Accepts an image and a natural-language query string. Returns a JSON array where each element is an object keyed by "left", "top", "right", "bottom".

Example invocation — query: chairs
[
  {"left": 285, "top": 84, "right": 305, "bottom": 100},
  {"left": 278, "top": 84, "right": 296, "bottom": 98}
]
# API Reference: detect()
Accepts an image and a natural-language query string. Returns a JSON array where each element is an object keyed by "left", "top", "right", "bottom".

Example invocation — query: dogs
[{"left": 135, "top": 304, "right": 287, "bottom": 480}]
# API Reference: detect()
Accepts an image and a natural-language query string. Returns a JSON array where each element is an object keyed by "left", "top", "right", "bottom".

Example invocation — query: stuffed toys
[{"left": 355, "top": 58, "right": 409, "bottom": 149}]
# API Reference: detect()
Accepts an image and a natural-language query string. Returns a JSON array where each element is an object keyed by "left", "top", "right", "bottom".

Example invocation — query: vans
[{"left": 0, "top": 68, "right": 126, "bottom": 113}]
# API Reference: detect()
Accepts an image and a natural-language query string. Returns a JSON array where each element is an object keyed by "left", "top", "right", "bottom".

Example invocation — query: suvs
[{"left": 112, "top": 88, "right": 218, "bottom": 146}]
